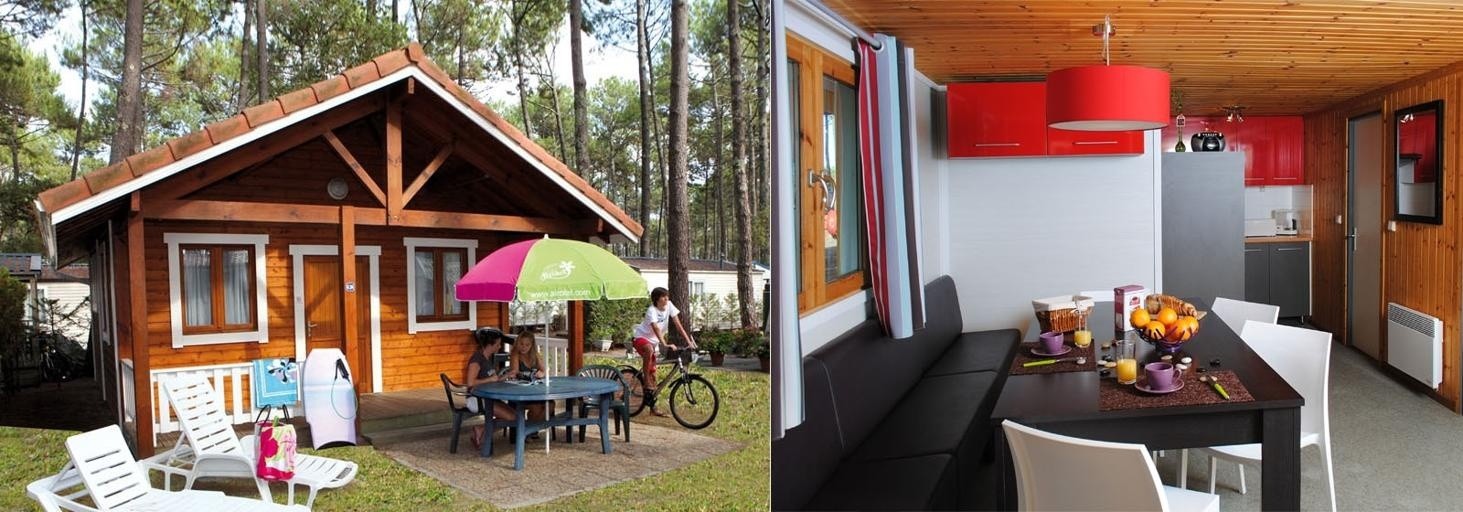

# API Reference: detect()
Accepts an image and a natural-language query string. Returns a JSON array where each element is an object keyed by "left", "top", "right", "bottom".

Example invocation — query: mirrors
[{"left": 1394, "top": 99, "right": 1443, "bottom": 226}]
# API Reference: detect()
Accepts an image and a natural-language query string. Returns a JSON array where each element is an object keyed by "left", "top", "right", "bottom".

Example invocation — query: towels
[{"left": 251, "top": 355, "right": 298, "bottom": 408}]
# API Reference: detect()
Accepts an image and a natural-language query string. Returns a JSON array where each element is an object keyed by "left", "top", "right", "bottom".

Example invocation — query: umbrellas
[{"left": 454, "top": 232, "right": 650, "bottom": 454}]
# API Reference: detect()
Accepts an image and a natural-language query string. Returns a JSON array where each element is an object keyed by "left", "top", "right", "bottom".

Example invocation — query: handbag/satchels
[{"left": 254, "top": 404, "right": 296, "bottom": 482}]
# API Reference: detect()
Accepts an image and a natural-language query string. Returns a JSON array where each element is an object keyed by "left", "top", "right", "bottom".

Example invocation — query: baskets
[
  {"left": 1132, "top": 320, "right": 1200, "bottom": 366},
  {"left": 1032, "top": 294, "right": 1094, "bottom": 334}
]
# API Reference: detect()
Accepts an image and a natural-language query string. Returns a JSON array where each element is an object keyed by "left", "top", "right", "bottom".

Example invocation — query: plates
[
  {"left": 1134, "top": 377, "right": 1184, "bottom": 393},
  {"left": 1030, "top": 346, "right": 1071, "bottom": 357}
]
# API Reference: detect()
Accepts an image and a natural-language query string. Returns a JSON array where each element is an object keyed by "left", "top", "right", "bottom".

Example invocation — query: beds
[{"left": 469, "top": 375, "right": 620, "bottom": 471}]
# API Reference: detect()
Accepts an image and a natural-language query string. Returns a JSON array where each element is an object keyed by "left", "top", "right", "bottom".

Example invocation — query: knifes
[
  {"left": 1023, "top": 356, "right": 1087, "bottom": 368},
  {"left": 1203, "top": 373, "right": 1229, "bottom": 400}
]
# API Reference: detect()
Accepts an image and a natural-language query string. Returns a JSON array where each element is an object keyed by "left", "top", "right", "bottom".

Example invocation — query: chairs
[
  {"left": 1151, "top": 297, "right": 1280, "bottom": 496},
  {"left": 1080, "top": 290, "right": 1116, "bottom": 302},
  {"left": 137, "top": 373, "right": 359, "bottom": 509},
  {"left": 499, "top": 366, "right": 556, "bottom": 442},
  {"left": 23, "top": 423, "right": 312, "bottom": 512},
  {"left": 1175, "top": 321, "right": 1337, "bottom": 512},
  {"left": 440, "top": 374, "right": 494, "bottom": 455},
  {"left": 1000, "top": 420, "right": 1221, "bottom": 512},
  {"left": 576, "top": 364, "right": 631, "bottom": 443}
]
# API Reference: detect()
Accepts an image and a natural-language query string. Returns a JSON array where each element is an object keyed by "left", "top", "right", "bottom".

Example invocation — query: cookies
[{"left": 1146, "top": 295, "right": 1197, "bottom": 318}]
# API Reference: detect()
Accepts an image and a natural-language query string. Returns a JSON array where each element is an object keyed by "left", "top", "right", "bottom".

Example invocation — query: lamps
[
  {"left": 1044, "top": 15, "right": 1171, "bottom": 132},
  {"left": 1222, "top": 106, "right": 1245, "bottom": 123}
]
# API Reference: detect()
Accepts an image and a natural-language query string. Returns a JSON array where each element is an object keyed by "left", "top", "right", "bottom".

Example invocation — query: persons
[
  {"left": 465, "top": 326, "right": 516, "bottom": 448},
  {"left": 631, "top": 287, "right": 696, "bottom": 416},
  {"left": 509, "top": 331, "right": 555, "bottom": 441}
]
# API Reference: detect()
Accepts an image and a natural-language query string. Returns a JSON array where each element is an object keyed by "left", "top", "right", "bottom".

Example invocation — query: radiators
[{"left": 1387, "top": 302, "right": 1444, "bottom": 390}]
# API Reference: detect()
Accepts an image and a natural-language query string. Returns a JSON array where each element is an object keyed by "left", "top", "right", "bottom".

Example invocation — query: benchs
[{"left": 772, "top": 275, "right": 1021, "bottom": 512}]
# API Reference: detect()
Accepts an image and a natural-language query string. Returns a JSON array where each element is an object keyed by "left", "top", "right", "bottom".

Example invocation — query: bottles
[
  {"left": 1173, "top": 125, "right": 1186, "bottom": 153},
  {"left": 530, "top": 368, "right": 538, "bottom": 384}
]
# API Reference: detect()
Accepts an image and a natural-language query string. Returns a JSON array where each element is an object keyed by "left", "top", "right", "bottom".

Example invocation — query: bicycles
[
  {"left": 40, "top": 327, "right": 58, "bottom": 382},
  {"left": 610, "top": 343, "right": 719, "bottom": 430}
]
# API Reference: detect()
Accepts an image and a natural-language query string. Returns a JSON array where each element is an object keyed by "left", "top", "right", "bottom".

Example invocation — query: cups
[
  {"left": 1115, "top": 339, "right": 1136, "bottom": 385},
  {"left": 1038, "top": 332, "right": 1064, "bottom": 352},
  {"left": 1072, "top": 295, "right": 1095, "bottom": 348},
  {"left": 1144, "top": 361, "right": 1181, "bottom": 389}
]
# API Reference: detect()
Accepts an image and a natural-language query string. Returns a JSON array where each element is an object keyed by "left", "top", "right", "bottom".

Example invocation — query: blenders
[{"left": 1274, "top": 208, "right": 1298, "bottom": 235}]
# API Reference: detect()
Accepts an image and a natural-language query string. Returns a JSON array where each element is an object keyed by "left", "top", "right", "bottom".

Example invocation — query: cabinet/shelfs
[
  {"left": 1245, "top": 242, "right": 1310, "bottom": 324},
  {"left": 1161, "top": 114, "right": 1236, "bottom": 152},
  {"left": 946, "top": 82, "right": 1145, "bottom": 159},
  {"left": 1237, "top": 116, "right": 1304, "bottom": 185}
]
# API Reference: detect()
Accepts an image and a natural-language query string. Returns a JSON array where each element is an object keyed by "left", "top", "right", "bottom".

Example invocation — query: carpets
[{"left": 359, "top": 387, "right": 750, "bottom": 511}]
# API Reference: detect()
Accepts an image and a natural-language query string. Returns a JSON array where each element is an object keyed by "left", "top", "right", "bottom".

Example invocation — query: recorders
[{"left": 1191, "top": 130, "right": 1225, "bottom": 152}]
